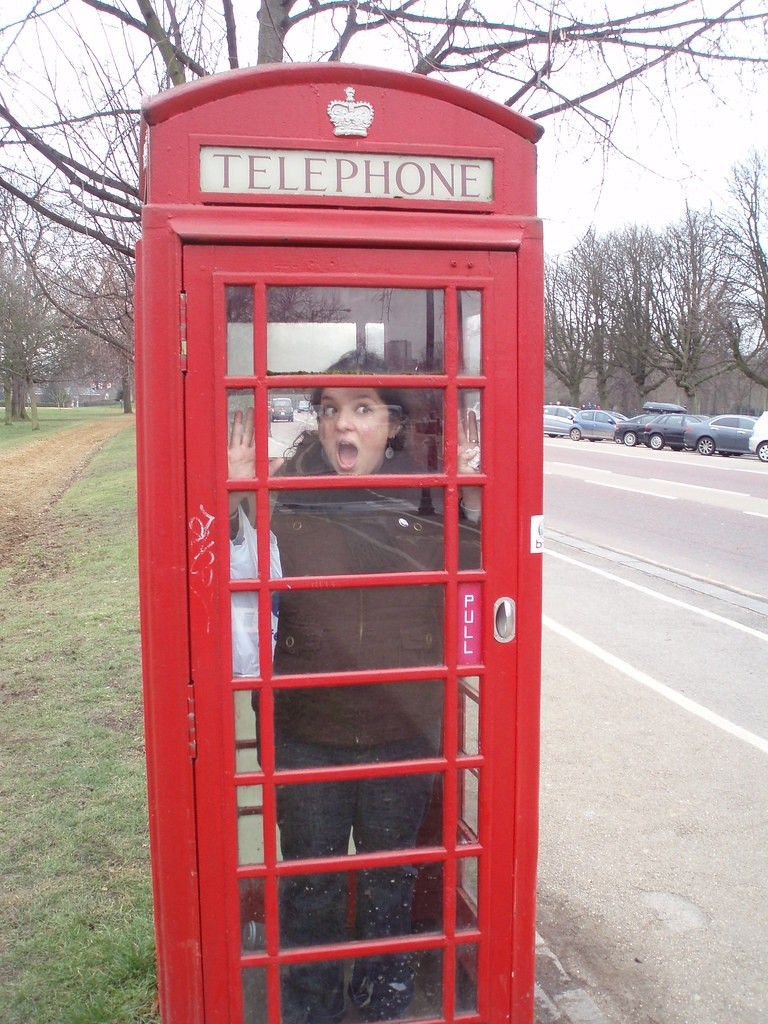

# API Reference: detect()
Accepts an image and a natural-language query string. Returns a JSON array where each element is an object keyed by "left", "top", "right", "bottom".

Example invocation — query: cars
[
  {"left": 467, "top": 402, "right": 768, "bottom": 462},
  {"left": 298, "top": 400, "right": 313, "bottom": 413}
]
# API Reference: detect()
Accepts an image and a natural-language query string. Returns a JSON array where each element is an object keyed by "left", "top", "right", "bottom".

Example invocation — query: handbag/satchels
[{"left": 230, "top": 503, "right": 282, "bottom": 678}]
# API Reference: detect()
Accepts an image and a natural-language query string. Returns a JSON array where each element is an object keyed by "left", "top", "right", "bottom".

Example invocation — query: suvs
[{"left": 272, "top": 398, "right": 293, "bottom": 422}]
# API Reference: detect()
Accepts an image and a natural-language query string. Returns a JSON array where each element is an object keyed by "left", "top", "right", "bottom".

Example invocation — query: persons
[{"left": 228, "top": 351, "right": 483, "bottom": 1023}]
